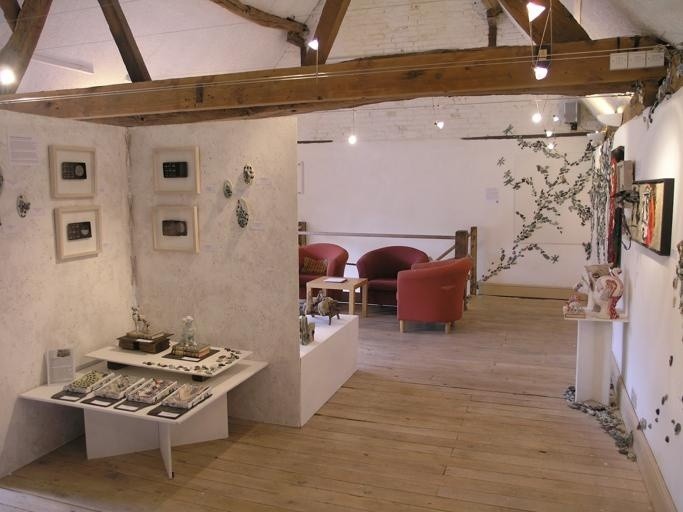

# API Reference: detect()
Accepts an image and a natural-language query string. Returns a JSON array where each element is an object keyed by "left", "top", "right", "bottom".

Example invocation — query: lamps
[{"left": 308, "top": 0, "right": 563, "bottom": 149}]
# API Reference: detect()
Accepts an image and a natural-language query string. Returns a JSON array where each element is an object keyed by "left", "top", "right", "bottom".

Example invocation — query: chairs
[
  {"left": 356, "top": 246, "right": 428, "bottom": 308},
  {"left": 299, "top": 243, "right": 348, "bottom": 300},
  {"left": 397, "top": 257, "right": 472, "bottom": 334}
]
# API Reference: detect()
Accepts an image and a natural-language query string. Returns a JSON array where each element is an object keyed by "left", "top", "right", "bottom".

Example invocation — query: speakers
[{"left": 564, "top": 101, "right": 578, "bottom": 123}]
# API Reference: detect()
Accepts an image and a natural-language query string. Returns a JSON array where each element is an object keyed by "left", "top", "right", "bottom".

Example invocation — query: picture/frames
[
  {"left": 616, "top": 160, "right": 634, "bottom": 209},
  {"left": 627, "top": 179, "right": 673, "bottom": 256},
  {"left": 48, "top": 145, "right": 201, "bottom": 261}
]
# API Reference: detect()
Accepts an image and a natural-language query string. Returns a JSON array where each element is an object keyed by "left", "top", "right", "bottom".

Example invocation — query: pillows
[
  {"left": 301, "top": 256, "right": 328, "bottom": 274},
  {"left": 367, "top": 263, "right": 411, "bottom": 281}
]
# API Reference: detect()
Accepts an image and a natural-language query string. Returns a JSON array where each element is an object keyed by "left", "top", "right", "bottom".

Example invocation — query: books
[
  {"left": 171, "top": 341, "right": 210, "bottom": 358},
  {"left": 128, "top": 328, "right": 164, "bottom": 339}
]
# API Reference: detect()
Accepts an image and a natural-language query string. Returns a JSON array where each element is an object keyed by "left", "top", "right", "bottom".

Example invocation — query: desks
[
  {"left": 18, "top": 340, "right": 268, "bottom": 479},
  {"left": 563, "top": 304, "right": 628, "bottom": 408}
]
